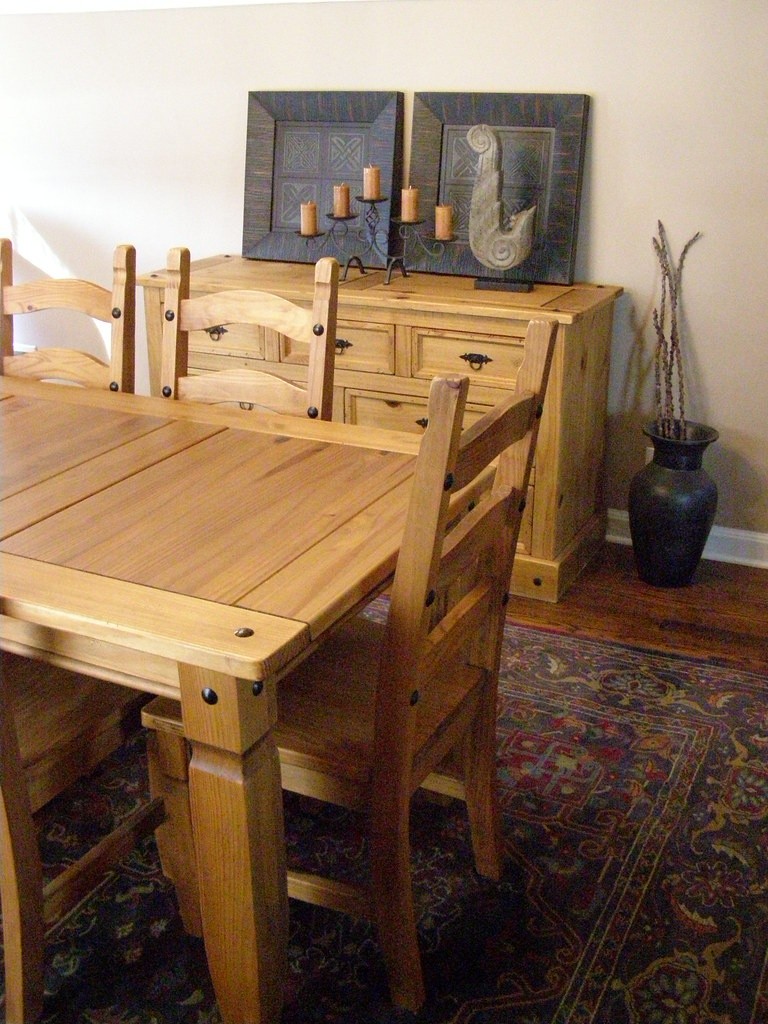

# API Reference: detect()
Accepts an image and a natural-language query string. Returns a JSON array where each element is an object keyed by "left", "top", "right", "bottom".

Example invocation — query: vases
[{"left": 627, "top": 420, "right": 720, "bottom": 589}]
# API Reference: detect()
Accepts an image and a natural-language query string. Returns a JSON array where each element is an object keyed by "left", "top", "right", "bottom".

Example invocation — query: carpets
[{"left": 0, "top": 593, "right": 768, "bottom": 1024}]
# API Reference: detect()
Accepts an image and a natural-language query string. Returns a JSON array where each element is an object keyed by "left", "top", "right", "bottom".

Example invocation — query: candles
[
  {"left": 333, "top": 182, "right": 350, "bottom": 218},
  {"left": 300, "top": 201, "right": 317, "bottom": 236},
  {"left": 434, "top": 205, "right": 452, "bottom": 240},
  {"left": 364, "top": 163, "right": 381, "bottom": 199},
  {"left": 406, "top": 185, "right": 419, "bottom": 226}
]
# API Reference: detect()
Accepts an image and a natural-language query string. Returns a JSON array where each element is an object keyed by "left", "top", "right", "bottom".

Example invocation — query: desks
[{"left": 0, "top": 377, "right": 499, "bottom": 1024}]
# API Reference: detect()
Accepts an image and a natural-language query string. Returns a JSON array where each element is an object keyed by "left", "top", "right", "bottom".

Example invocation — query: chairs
[
  {"left": 160, "top": 246, "right": 340, "bottom": 421},
  {"left": 0, "top": 238, "right": 137, "bottom": 394},
  {"left": 141, "top": 319, "right": 560, "bottom": 1014},
  {"left": 0, "top": 595, "right": 202, "bottom": 1024}
]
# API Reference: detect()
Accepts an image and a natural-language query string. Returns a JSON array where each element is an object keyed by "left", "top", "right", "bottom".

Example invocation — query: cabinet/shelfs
[{"left": 137, "top": 252, "right": 625, "bottom": 608}]
